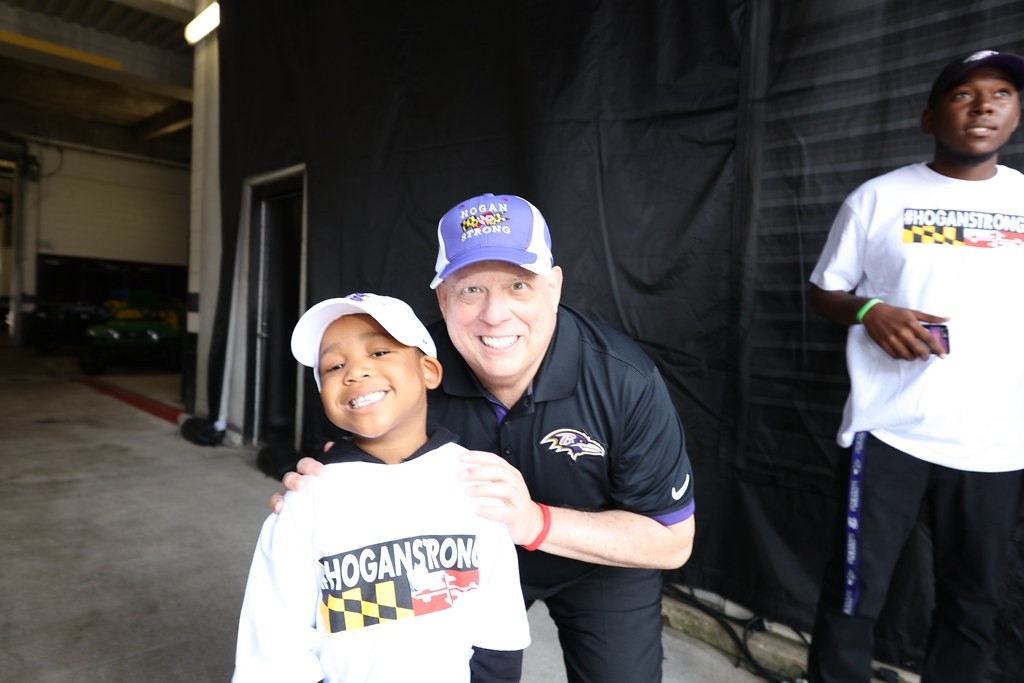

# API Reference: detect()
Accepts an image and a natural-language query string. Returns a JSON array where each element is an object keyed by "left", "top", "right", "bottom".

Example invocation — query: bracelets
[
  {"left": 520, "top": 502, "right": 551, "bottom": 552},
  {"left": 856, "top": 297, "right": 885, "bottom": 324}
]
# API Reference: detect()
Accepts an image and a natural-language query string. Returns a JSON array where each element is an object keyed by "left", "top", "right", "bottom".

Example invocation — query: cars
[{"left": 84, "top": 309, "right": 185, "bottom": 378}]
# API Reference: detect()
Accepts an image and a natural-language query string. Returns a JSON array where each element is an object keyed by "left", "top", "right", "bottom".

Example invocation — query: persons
[
  {"left": 269, "top": 194, "right": 695, "bottom": 683},
  {"left": 806, "top": 49, "right": 1023, "bottom": 681},
  {"left": 232, "top": 292, "right": 529, "bottom": 682}
]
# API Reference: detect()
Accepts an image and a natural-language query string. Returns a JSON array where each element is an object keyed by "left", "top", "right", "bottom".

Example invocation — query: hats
[
  {"left": 429, "top": 192, "right": 554, "bottom": 291},
  {"left": 290, "top": 292, "right": 438, "bottom": 394},
  {"left": 927, "top": 49, "right": 1024, "bottom": 111}
]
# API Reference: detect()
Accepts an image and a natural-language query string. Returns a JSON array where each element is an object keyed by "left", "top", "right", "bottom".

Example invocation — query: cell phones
[{"left": 916, "top": 325, "right": 950, "bottom": 355}]
